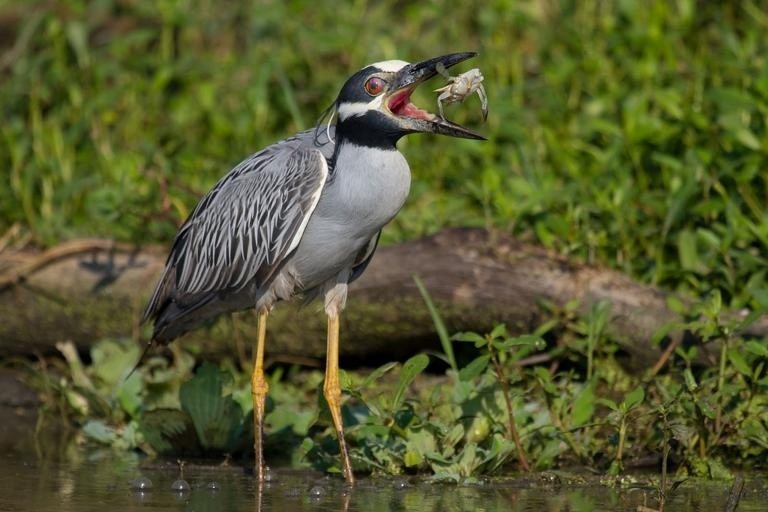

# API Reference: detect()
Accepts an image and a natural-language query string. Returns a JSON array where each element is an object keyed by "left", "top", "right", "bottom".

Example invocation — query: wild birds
[{"left": 125, "top": 51, "right": 491, "bottom": 488}]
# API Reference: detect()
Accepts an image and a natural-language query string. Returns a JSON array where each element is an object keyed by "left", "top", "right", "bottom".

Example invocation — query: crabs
[{"left": 431, "top": 67, "right": 489, "bottom": 126}]
